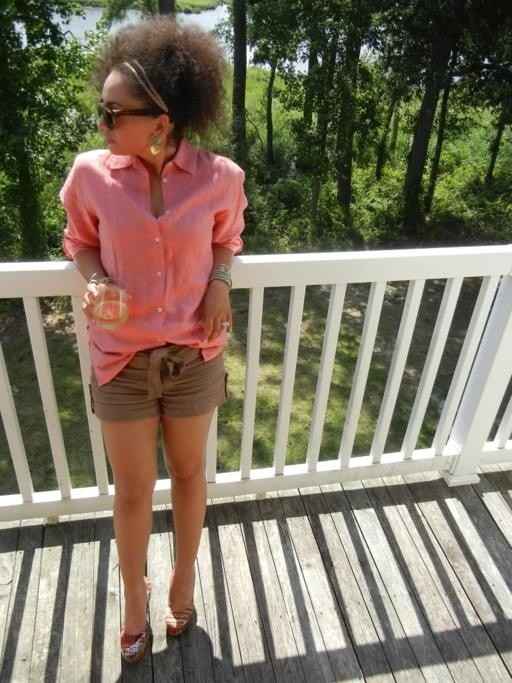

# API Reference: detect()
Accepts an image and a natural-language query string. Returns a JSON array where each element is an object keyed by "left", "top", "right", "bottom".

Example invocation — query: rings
[{"left": 222, "top": 320, "right": 228, "bottom": 326}]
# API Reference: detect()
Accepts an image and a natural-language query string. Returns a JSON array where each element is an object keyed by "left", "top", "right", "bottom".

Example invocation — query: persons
[{"left": 59, "top": 14, "right": 249, "bottom": 664}]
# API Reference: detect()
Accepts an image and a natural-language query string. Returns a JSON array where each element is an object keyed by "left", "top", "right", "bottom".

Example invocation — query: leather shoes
[
  {"left": 165, "top": 560, "right": 195, "bottom": 635},
  {"left": 121, "top": 576, "right": 152, "bottom": 663}
]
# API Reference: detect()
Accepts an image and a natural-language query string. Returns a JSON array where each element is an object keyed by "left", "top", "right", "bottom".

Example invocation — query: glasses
[{"left": 97, "top": 102, "right": 163, "bottom": 128}]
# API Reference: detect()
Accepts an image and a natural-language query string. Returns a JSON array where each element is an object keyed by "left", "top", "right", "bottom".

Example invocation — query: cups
[{"left": 91, "top": 276, "right": 130, "bottom": 330}]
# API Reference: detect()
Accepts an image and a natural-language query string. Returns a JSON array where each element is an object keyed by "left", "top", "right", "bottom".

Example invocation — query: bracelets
[
  {"left": 86, "top": 270, "right": 106, "bottom": 284},
  {"left": 208, "top": 269, "right": 233, "bottom": 288}
]
